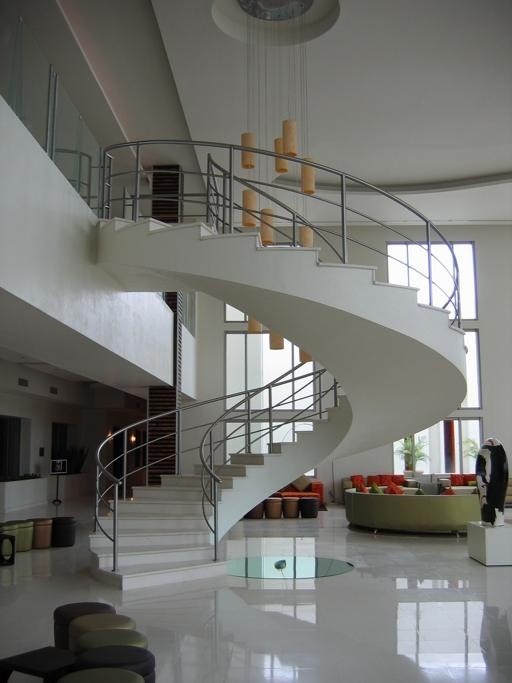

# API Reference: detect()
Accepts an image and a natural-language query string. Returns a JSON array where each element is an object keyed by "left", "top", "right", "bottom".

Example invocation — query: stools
[
  {"left": 246, "top": 497, "right": 318, "bottom": 519},
  {"left": 1, "top": 517, "right": 77, "bottom": 566},
  {"left": 1, "top": 601, "right": 157, "bottom": 683}
]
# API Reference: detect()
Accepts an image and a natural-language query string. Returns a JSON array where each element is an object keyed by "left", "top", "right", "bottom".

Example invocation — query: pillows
[
  {"left": 349, "top": 473, "right": 477, "bottom": 487},
  {"left": 291, "top": 473, "right": 309, "bottom": 491}
]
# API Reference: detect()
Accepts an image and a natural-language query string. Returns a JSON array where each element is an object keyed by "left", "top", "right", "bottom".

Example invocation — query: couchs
[
  {"left": 342, "top": 478, "right": 512, "bottom": 542},
  {"left": 272, "top": 478, "right": 324, "bottom": 509}
]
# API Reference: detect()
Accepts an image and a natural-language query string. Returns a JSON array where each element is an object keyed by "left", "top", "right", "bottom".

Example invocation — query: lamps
[{"left": 239, "top": 0, "right": 317, "bottom": 362}]
